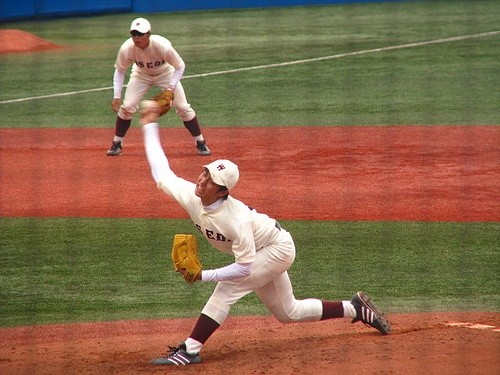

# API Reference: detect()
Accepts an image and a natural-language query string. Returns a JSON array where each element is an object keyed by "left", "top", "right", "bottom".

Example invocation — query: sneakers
[
  {"left": 107, "top": 140, "right": 123, "bottom": 155},
  {"left": 350, "top": 289, "right": 392, "bottom": 335},
  {"left": 149, "top": 343, "right": 203, "bottom": 366},
  {"left": 196, "top": 137, "right": 211, "bottom": 156}
]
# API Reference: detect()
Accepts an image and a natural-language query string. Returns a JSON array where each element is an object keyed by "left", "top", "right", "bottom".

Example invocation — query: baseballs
[{"left": 142, "top": 100, "right": 157, "bottom": 110}]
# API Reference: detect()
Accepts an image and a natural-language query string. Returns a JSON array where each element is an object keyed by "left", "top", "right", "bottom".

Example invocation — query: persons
[
  {"left": 105, "top": 17, "right": 210, "bottom": 155},
  {"left": 138, "top": 99, "right": 390, "bottom": 365}
]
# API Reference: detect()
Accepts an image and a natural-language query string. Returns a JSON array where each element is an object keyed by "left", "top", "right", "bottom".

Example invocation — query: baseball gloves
[
  {"left": 152, "top": 90, "right": 174, "bottom": 118},
  {"left": 171, "top": 233, "right": 202, "bottom": 284}
]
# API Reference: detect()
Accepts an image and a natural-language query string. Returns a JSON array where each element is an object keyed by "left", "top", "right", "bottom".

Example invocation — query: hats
[
  {"left": 130, "top": 19, "right": 152, "bottom": 33},
  {"left": 202, "top": 158, "right": 240, "bottom": 189}
]
